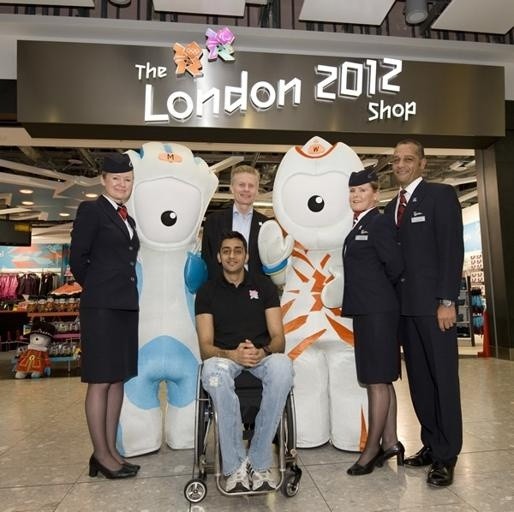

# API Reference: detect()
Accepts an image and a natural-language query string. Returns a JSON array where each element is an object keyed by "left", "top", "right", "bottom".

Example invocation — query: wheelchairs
[{"left": 183, "top": 356, "right": 299, "bottom": 503}]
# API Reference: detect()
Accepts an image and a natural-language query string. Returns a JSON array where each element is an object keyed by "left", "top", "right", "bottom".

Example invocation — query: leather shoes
[
  {"left": 426, "top": 455, "right": 457, "bottom": 488},
  {"left": 404, "top": 446, "right": 433, "bottom": 468}
]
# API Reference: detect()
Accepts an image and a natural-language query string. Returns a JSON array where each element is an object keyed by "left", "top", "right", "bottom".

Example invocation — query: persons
[
  {"left": 200, "top": 165, "right": 271, "bottom": 280},
  {"left": 67, "top": 153, "right": 141, "bottom": 479},
  {"left": 193, "top": 229, "right": 295, "bottom": 494},
  {"left": 383, "top": 139, "right": 465, "bottom": 486},
  {"left": 341, "top": 169, "right": 405, "bottom": 477}
]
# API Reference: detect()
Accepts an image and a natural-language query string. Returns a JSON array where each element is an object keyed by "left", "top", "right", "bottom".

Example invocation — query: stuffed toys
[
  {"left": 257, "top": 133, "right": 371, "bottom": 453},
  {"left": 13, "top": 322, "right": 56, "bottom": 379},
  {"left": 117, "top": 141, "right": 219, "bottom": 459}
]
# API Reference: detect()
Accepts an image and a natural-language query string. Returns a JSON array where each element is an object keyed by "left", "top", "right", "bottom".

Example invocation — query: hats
[
  {"left": 103, "top": 153, "right": 134, "bottom": 174},
  {"left": 349, "top": 168, "right": 378, "bottom": 186}
]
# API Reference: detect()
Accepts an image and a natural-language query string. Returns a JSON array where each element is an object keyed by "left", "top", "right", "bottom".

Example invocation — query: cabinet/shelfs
[{"left": 22, "top": 311, "right": 81, "bottom": 375}]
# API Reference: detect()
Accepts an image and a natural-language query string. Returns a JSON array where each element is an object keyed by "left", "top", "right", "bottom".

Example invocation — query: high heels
[
  {"left": 348, "top": 446, "right": 383, "bottom": 475},
  {"left": 89, "top": 455, "right": 141, "bottom": 479},
  {"left": 375, "top": 442, "right": 405, "bottom": 467}
]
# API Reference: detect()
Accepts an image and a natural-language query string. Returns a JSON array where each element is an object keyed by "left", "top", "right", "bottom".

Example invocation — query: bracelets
[{"left": 262, "top": 346, "right": 272, "bottom": 357}]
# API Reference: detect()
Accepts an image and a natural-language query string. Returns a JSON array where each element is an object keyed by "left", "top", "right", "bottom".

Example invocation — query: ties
[{"left": 397, "top": 190, "right": 407, "bottom": 227}]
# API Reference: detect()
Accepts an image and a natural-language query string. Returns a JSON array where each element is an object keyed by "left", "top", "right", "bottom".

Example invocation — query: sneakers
[
  {"left": 248, "top": 460, "right": 277, "bottom": 491},
  {"left": 226, "top": 457, "right": 251, "bottom": 493}
]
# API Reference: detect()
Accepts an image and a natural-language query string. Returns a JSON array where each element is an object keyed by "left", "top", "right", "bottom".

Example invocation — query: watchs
[{"left": 439, "top": 298, "right": 457, "bottom": 306}]
